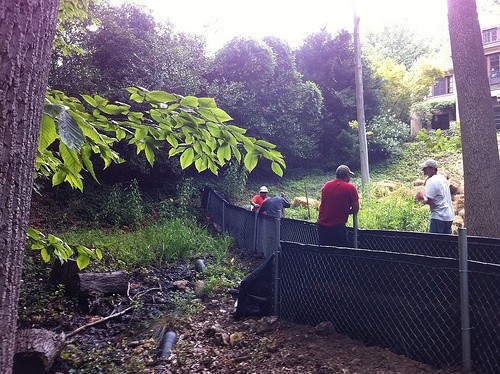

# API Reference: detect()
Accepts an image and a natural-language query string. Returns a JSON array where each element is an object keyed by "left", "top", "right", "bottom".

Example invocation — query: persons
[
  {"left": 414, "top": 158, "right": 455, "bottom": 234},
  {"left": 259, "top": 187, "right": 290, "bottom": 218},
  {"left": 318, "top": 165, "right": 360, "bottom": 247},
  {"left": 250, "top": 186, "right": 269, "bottom": 215}
]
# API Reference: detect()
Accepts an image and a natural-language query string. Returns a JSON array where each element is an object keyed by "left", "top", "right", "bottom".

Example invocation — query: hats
[
  {"left": 260, "top": 186, "right": 268, "bottom": 193},
  {"left": 419, "top": 159, "right": 437, "bottom": 168},
  {"left": 336, "top": 165, "right": 354, "bottom": 174}
]
